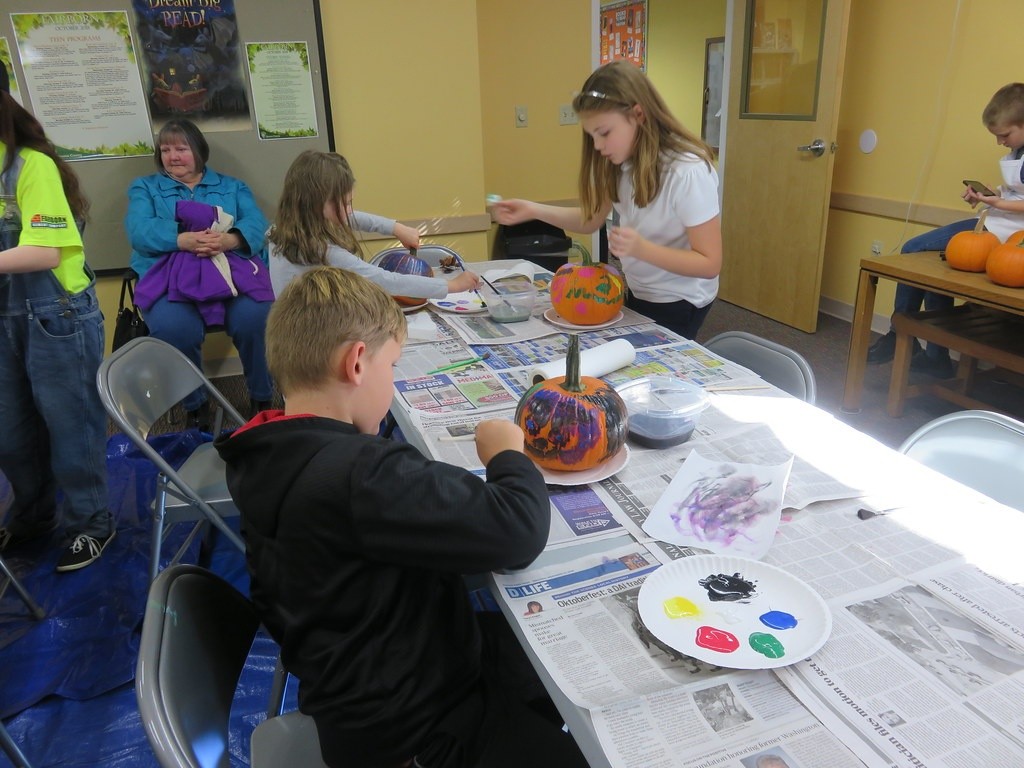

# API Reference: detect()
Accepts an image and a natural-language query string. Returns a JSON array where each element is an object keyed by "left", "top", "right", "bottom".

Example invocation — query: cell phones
[{"left": 963, "top": 181, "right": 995, "bottom": 196}]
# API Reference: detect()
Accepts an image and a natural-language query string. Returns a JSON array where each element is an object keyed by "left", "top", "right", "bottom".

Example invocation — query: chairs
[
  {"left": 94, "top": 336, "right": 329, "bottom": 768},
  {"left": 897, "top": 410, "right": 1024, "bottom": 517},
  {"left": 703, "top": 330, "right": 815, "bottom": 409},
  {"left": 368, "top": 245, "right": 464, "bottom": 267},
  {"left": 123, "top": 267, "right": 226, "bottom": 424}
]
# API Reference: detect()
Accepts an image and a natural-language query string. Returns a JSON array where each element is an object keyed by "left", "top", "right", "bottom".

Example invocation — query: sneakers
[
  {"left": 0, "top": 517, "right": 61, "bottom": 552},
  {"left": 56, "top": 529, "right": 115, "bottom": 572}
]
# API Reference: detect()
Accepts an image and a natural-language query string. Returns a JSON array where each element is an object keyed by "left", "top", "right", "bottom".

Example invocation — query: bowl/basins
[
  {"left": 480, "top": 282, "right": 537, "bottom": 322},
  {"left": 613, "top": 375, "right": 710, "bottom": 448}
]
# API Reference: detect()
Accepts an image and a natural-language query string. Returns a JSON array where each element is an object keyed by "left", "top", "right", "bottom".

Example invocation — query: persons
[
  {"left": 632, "top": 616, "right": 698, "bottom": 673},
  {"left": 868, "top": 83, "right": 1024, "bottom": 379},
  {"left": 126, "top": 120, "right": 274, "bottom": 432},
  {"left": 757, "top": 755, "right": 789, "bottom": 768},
  {"left": 455, "top": 425, "right": 473, "bottom": 435},
  {"left": 525, "top": 601, "right": 543, "bottom": 615},
  {"left": 0, "top": 88, "right": 116, "bottom": 570},
  {"left": 696, "top": 687, "right": 752, "bottom": 728},
  {"left": 212, "top": 267, "right": 591, "bottom": 768},
  {"left": 494, "top": 60, "right": 722, "bottom": 342},
  {"left": 881, "top": 713, "right": 903, "bottom": 725},
  {"left": 269, "top": 149, "right": 480, "bottom": 302}
]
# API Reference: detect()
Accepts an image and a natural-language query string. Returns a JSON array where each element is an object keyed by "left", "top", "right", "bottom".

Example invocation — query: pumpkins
[{"left": 945, "top": 209, "right": 1024, "bottom": 287}]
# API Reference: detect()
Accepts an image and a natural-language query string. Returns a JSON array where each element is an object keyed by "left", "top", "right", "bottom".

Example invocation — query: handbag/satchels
[{"left": 112, "top": 268, "right": 150, "bottom": 354}]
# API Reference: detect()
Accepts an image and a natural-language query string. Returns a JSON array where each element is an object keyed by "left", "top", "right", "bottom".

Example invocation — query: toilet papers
[{"left": 529, "top": 337, "right": 636, "bottom": 389}]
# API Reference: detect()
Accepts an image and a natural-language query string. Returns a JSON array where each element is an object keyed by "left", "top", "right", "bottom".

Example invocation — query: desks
[
  {"left": 839, "top": 249, "right": 1024, "bottom": 418},
  {"left": 389, "top": 259, "right": 1024, "bottom": 768}
]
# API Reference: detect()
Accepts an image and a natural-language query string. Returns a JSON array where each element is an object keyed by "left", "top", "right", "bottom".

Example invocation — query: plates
[
  {"left": 638, "top": 554, "right": 832, "bottom": 669},
  {"left": 430, "top": 292, "right": 500, "bottom": 313},
  {"left": 544, "top": 307, "right": 624, "bottom": 329},
  {"left": 531, "top": 440, "right": 630, "bottom": 485},
  {"left": 399, "top": 302, "right": 428, "bottom": 311}
]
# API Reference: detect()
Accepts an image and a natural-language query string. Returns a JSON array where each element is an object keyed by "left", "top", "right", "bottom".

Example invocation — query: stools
[{"left": 885, "top": 300, "right": 1024, "bottom": 417}]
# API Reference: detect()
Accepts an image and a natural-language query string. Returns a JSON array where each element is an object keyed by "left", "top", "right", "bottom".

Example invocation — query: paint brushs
[
  {"left": 656, "top": 386, "right": 770, "bottom": 394},
  {"left": 426, "top": 353, "right": 491, "bottom": 375},
  {"left": 436, "top": 434, "right": 475, "bottom": 442},
  {"left": 454, "top": 253, "right": 487, "bottom": 307},
  {"left": 481, "top": 275, "right": 520, "bottom": 314}
]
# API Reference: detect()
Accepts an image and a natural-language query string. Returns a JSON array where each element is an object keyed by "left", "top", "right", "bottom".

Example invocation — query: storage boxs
[
  {"left": 613, "top": 377, "right": 710, "bottom": 449},
  {"left": 481, "top": 282, "right": 537, "bottom": 322}
]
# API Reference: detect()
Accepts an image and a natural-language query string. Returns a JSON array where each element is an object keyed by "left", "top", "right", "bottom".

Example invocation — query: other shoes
[
  {"left": 185, "top": 401, "right": 210, "bottom": 432},
  {"left": 249, "top": 401, "right": 272, "bottom": 421},
  {"left": 911, "top": 349, "right": 953, "bottom": 380},
  {"left": 866, "top": 332, "right": 921, "bottom": 364}
]
